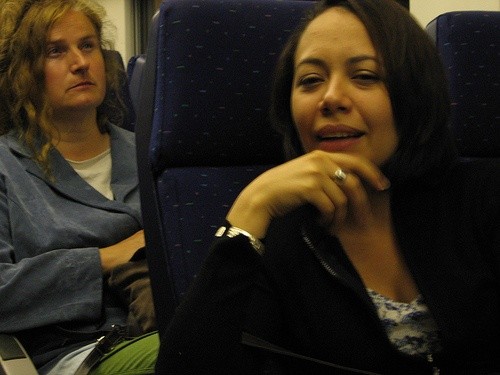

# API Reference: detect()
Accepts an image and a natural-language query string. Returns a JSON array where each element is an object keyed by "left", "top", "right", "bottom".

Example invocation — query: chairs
[{"left": 106, "top": 0, "right": 500, "bottom": 336}]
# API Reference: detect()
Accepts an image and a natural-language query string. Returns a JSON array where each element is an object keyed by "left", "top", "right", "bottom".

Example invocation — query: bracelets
[{"left": 214, "top": 226, "right": 264, "bottom": 258}]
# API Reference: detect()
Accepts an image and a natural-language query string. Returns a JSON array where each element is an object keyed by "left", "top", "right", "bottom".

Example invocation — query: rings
[{"left": 334, "top": 168, "right": 348, "bottom": 187}]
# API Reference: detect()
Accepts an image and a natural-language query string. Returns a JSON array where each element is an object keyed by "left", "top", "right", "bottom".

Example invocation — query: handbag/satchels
[{"left": 104, "top": 257, "right": 159, "bottom": 342}]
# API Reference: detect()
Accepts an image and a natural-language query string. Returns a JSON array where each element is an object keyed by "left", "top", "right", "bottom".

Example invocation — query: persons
[
  {"left": 153, "top": 1, "right": 500, "bottom": 375},
  {"left": 0, "top": 1, "right": 161, "bottom": 374}
]
